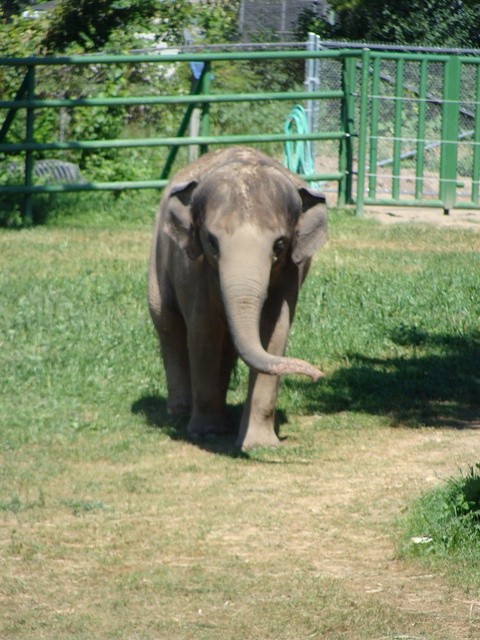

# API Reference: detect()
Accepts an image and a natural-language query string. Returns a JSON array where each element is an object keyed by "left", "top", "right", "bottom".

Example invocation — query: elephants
[{"left": 144, "top": 141, "right": 331, "bottom": 457}]
